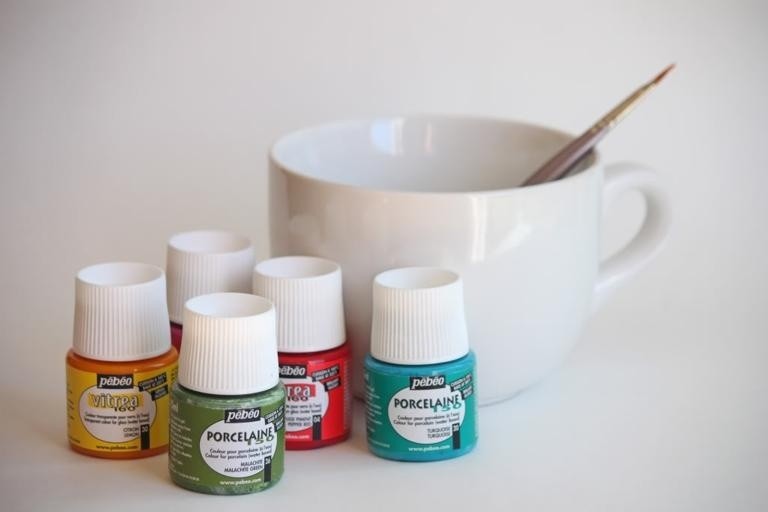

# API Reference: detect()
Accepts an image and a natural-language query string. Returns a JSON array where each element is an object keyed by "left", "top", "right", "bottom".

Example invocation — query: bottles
[
  {"left": 165, "top": 230, "right": 258, "bottom": 353},
  {"left": 253, "top": 253, "right": 354, "bottom": 450},
  {"left": 366, "top": 267, "right": 477, "bottom": 461},
  {"left": 66, "top": 260, "right": 177, "bottom": 458},
  {"left": 170, "top": 292, "right": 286, "bottom": 495}
]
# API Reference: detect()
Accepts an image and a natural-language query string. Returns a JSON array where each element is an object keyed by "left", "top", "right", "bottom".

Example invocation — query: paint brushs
[{"left": 518, "top": 64, "right": 676, "bottom": 188}]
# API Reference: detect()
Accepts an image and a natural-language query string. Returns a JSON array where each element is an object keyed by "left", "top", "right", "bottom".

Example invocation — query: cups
[{"left": 262, "top": 109, "right": 678, "bottom": 413}]
